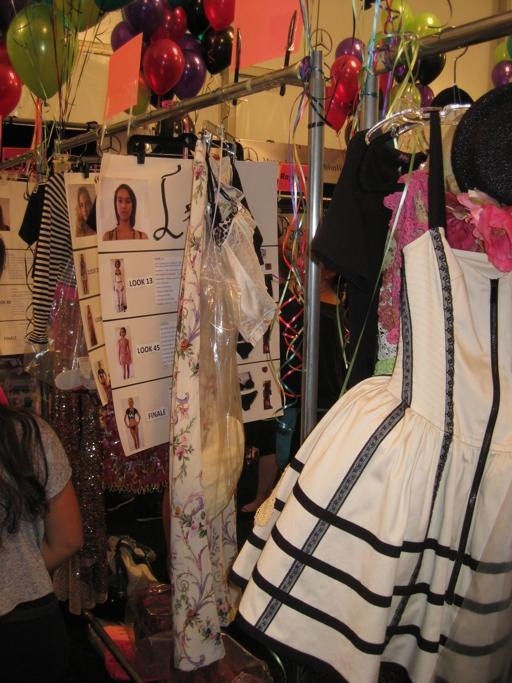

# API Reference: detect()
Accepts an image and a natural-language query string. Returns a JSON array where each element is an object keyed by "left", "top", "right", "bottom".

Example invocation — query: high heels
[{"left": 114, "top": 537, "right": 171, "bottom": 597}]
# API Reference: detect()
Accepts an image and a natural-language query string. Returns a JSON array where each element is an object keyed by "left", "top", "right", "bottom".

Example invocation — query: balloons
[
  {"left": 329, "top": 0, "right": 446, "bottom": 126},
  {"left": 492, "top": 35, "right": 512, "bottom": 88},
  {"left": 0, "top": 0, "right": 235, "bottom": 123}
]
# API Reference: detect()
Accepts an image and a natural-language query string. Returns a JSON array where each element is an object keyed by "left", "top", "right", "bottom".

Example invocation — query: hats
[{"left": 451, "top": 82, "right": 511, "bottom": 205}]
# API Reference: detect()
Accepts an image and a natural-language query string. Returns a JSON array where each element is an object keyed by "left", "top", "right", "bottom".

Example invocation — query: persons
[
  {"left": 124, "top": 397, "right": 141, "bottom": 449},
  {"left": 112, "top": 260, "right": 125, "bottom": 313},
  {"left": 74, "top": 186, "right": 97, "bottom": 238},
  {"left": 103, "top": 184, "right": 148, "bottom": 242},
  {"left": 97, "top": 361, "right": 109, "bottom": 399},
  {"left": 0, "top": 401, "right": 85, "bottom": 683},
  {"left": 118, "top": 327, "right": 132, "bottom": 379}
]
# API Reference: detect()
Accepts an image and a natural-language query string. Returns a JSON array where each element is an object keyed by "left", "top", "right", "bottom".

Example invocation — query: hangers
[{"left": 356, "top": 20, "right": 479, "bottom": 147}]
